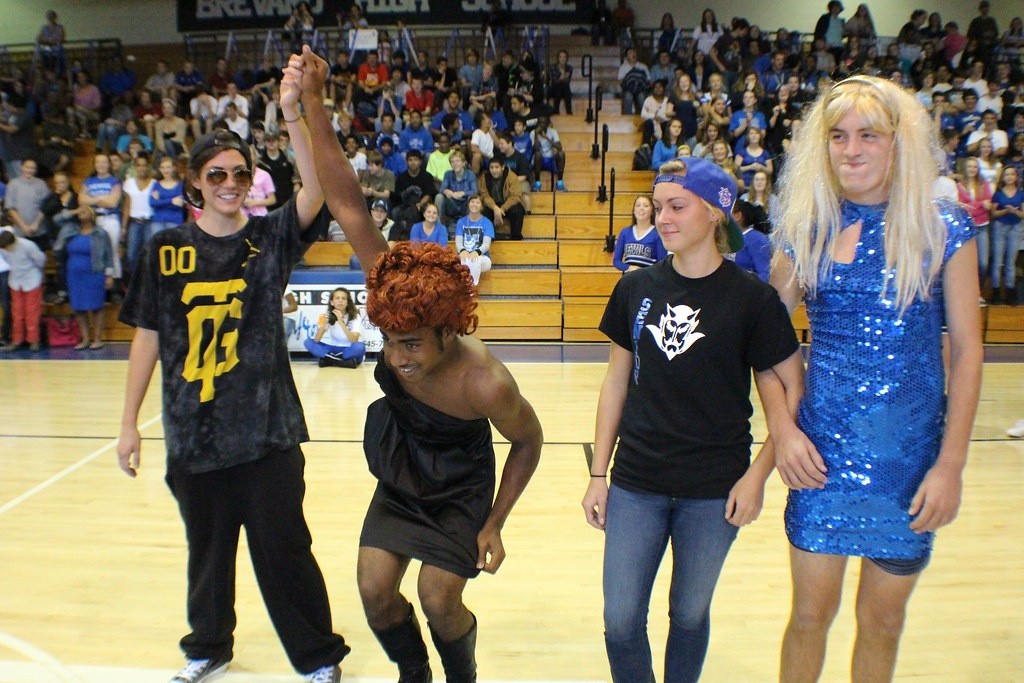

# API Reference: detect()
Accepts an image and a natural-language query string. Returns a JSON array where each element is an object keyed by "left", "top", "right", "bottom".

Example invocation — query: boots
[
  {"left": 371, "top": 605, "right": 432, "bottom": 683},
  {"left": 427, "top": 610, "right": 478, "bottom": 683},
  {"left": 1005, "top": 287, "right": 1018, "bottom": 305},
  {"left": 992, "top": 287, "right": 1002, "bottom": 305}
]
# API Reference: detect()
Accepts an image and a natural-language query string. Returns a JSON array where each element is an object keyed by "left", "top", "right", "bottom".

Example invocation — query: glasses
[
  {"left": 372, "top": 209, "right": 385, "bottom": 212},
  {"left": 196, "top": 169, "right": 252, "bottom": 188}
]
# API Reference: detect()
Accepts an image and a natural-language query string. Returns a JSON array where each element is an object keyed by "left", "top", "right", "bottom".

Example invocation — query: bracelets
[
  {"left": 590, "top": 473, "right": 607, "bottom": 478},
  {"left": 284, "top": 116, "right": 300, "bottom": 123}
]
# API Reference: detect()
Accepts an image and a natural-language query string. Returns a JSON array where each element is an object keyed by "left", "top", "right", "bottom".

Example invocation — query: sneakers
[
  {"left": 532, "top": 182, "right": 541, "bottom": 192},
  {"left": 556, "top": 180, "right": 567, "bottom": 192}
]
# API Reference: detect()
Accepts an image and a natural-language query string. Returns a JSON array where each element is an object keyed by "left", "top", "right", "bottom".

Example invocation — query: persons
[
  {"left": 0, "top": 0, "right": 572, "bottom": 352},
  {"left": 303, "top": 288, "right": 365, "bottom": 369},
  {"left": 755, "top": 74, "right": 980, "bottom": 683},
  {"left": 581, "top": 150, "right": 808, "bottom": 683},
  {"left": 115, "top": 53, "right": 351, "bottom": 683},
  {"left": 281, "top": 284, "right": 298, "bottom": 344},
  {"left": 301, "top": 44, "right": 543, "bottom": 683},
  {"left": 590, "top": 0, "right": 1024, "bottom": 436},
  {"left": 613, "top": 194, "right": 667, "bottom": 275}
]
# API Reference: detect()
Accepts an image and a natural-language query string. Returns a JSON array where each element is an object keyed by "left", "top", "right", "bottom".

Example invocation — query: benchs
[{"left": 0, "top": 28, "right": 1024, "bottom": 343}]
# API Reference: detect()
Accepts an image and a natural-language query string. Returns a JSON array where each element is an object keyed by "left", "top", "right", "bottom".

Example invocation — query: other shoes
[
  {"left": 91, "top": 340, "right": 103, "bottom": 349},
  {"left": 7, "top": 343, "right": 21, "bottom": 351},
  {"left": 511, "top": 234, "right": 521, "bottom": 239},
  {"left": 74, "top": 338, "right": 91, "bottom": 351},
  {"left": 30, "top": 342, "right": 38, "bottom": 352},
  {"left": 173, "top": 649, "right": 232, "bottom": 683},
  {"left": 319, "top": 356, "right": 329, "bottom": 367},
  {"left": 348, "top": 357, "right": 357, "bottom": 369},
  {"left": 305, "top": 663, "right": 341, "bottom": 683}
]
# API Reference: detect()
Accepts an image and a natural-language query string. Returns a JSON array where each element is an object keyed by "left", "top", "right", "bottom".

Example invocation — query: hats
[
  {"left": 653, "top": 160, "right": 745, "bottom": 251},
  {"left": 182, "top": 129, "right": 253, "bottom": 209},
  {"left": 372, "top": 200, "right": 387, "bottom": 212}
]
